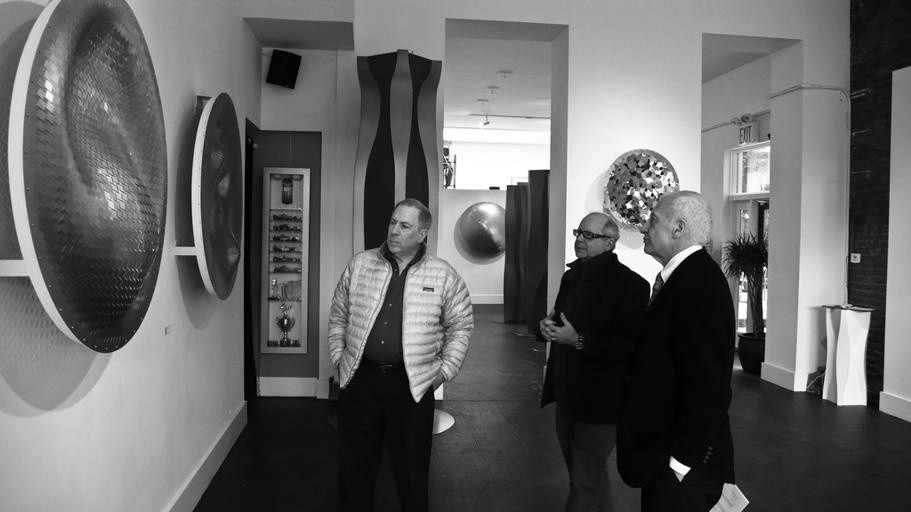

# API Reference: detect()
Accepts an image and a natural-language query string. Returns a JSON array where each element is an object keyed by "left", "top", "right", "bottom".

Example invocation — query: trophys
[{"left": 278, "top": 303, "right": 296, "bottom": 347}]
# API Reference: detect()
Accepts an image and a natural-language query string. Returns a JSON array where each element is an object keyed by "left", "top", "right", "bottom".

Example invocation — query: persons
[
  {"left": 617, "top": 189, "right": 736, "bottom": 512},
  {"left": 537, "top": 211, "right": 654, "bottom": 511},
  {"left": 327, "top": 197, "right": 476, "bottom": 511}
]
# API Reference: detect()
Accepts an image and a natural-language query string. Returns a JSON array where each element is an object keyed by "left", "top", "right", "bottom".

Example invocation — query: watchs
[{"left": 576, "top": 337, "right": 585, "bottom": 351}]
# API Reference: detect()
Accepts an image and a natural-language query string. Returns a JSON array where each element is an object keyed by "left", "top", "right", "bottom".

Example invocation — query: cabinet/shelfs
[{"left": 260, "top": 168, "right": 310, "bottom": 354}]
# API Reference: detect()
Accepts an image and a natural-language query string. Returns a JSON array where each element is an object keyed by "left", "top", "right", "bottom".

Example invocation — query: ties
[{"left": 647, "top": 271, "right": 664, "bottom": 306}]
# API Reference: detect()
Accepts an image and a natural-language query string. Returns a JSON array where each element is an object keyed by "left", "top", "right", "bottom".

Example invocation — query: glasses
[{"left": 572, "top": 229, "right": 612, "bottom": 240}]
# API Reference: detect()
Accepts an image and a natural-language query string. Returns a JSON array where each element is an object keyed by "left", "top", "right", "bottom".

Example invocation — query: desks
[{"left": 821, "top": 304, "right": 875, "bottom": 407}]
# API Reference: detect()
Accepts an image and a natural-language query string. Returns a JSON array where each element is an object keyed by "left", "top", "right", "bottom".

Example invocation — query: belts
[{"left": 365, "top": 363, "right": 399, "bottom": 372}]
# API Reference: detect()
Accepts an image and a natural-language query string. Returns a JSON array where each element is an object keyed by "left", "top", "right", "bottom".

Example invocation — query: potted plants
[{"left": 721, "top": 231, "right": 768, "bottom": 375}]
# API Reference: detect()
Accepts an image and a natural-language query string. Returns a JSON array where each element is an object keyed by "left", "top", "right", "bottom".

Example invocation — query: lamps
[{"left": 730, "top": 113, "right": 759, "bottom": 147}]
